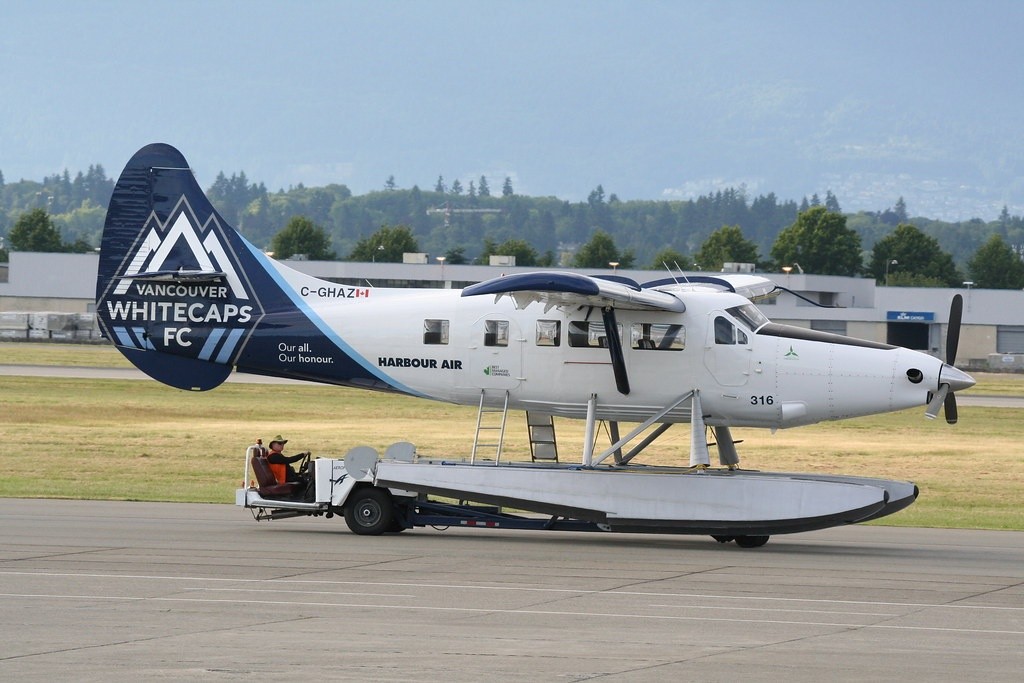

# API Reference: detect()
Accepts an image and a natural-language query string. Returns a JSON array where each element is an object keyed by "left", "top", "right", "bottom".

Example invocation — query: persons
[{"left": 266, "top": 434, "right": 311, "bottom": 495}]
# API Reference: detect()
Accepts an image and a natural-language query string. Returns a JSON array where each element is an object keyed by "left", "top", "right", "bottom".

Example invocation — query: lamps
[
  {"left": 609, "top": 263, "right": 619, "bottom": 275},
  {"left": 436, "top": 257, "right": 446, "bottom": 280},
  {"left": 783, "top": 267, "right": 792, "bottom": 287},
  {"left": 266, "top": 252, "right": 275, "bottom": 256}
]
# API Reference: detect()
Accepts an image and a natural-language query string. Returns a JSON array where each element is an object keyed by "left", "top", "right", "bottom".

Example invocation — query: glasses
[{"left": 274, "top": 442, "right": 284, "bottom": 445}]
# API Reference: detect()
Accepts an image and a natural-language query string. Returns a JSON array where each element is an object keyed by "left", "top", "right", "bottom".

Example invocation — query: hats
[{"left": 269, "top": 435, "right": 288, "bottom": 449}]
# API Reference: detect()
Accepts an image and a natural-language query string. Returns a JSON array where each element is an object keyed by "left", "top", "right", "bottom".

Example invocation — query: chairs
[
  {"left": 638, "top": 339, "right": 655, "bottom": 349},
  {"left": 251, "top": 448, "right": 301, "bottom": 497},
  {"left": 486, "top": 333, "right": 495, "bottom": 345},
  {"left": 425, "top": 332, "right": 441, "bottom": 344},
  {"left": 598, "top": 336, "right": 609, "bottom": 347}
]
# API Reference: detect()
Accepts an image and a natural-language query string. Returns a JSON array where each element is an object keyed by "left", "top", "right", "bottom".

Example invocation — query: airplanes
[{"left": 94, "top": 142, "right": 975, "bottom": 549}]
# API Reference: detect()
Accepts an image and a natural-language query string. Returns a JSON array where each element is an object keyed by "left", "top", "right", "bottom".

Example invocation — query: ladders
[{"left": 470, "top": 387, "right": 509, "bottom": 466}]
